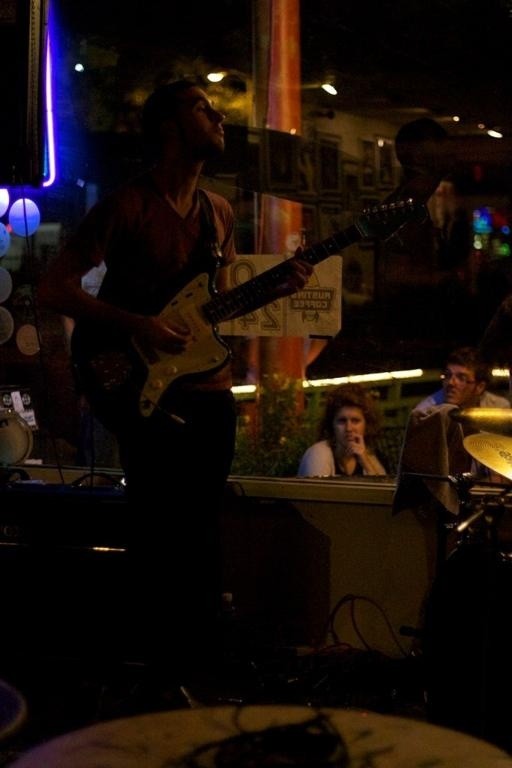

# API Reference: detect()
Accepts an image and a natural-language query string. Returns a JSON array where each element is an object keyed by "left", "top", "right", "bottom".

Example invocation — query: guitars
[{"left": 69, "top": 196, "right": 430, "bottom": 447}]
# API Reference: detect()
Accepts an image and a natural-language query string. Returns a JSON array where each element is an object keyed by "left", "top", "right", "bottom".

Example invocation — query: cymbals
[{"left": 463, "top": 433, "right": 512, "bottom": 480}]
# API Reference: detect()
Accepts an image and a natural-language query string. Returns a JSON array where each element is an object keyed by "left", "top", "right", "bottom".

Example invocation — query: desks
[{"left": 12, "top": 701, "right": 512, "bottom": 765}]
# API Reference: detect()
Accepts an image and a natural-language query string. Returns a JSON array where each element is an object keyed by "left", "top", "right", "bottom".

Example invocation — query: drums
[{"left": 465, "top": 475, "right": 512, "bottom": 562}]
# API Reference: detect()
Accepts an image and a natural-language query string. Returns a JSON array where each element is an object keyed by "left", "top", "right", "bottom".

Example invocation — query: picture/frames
[{"left": 301, "top": 126, "right": 403, "bottom": 261}]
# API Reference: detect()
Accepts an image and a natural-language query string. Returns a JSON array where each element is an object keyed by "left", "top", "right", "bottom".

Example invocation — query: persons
[
  {"left": 413, "top": 347, "right": 510, "bottom": 411},
  {"left": 33, "top": 81, "right": 313, "bottom": 708},
  {"left": 375, "top": 113, "right": 484, "bottom": 285},
  {"left": 430, "top": 166, "right": 476, "bottom": 272},
  {"left": 297, "top": 388, "right": 385, "bottom": 481}
]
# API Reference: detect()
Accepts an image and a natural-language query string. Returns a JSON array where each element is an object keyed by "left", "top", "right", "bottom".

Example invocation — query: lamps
[{"left": 318, "top": 72, "right": 341, "bottom": 96}]
